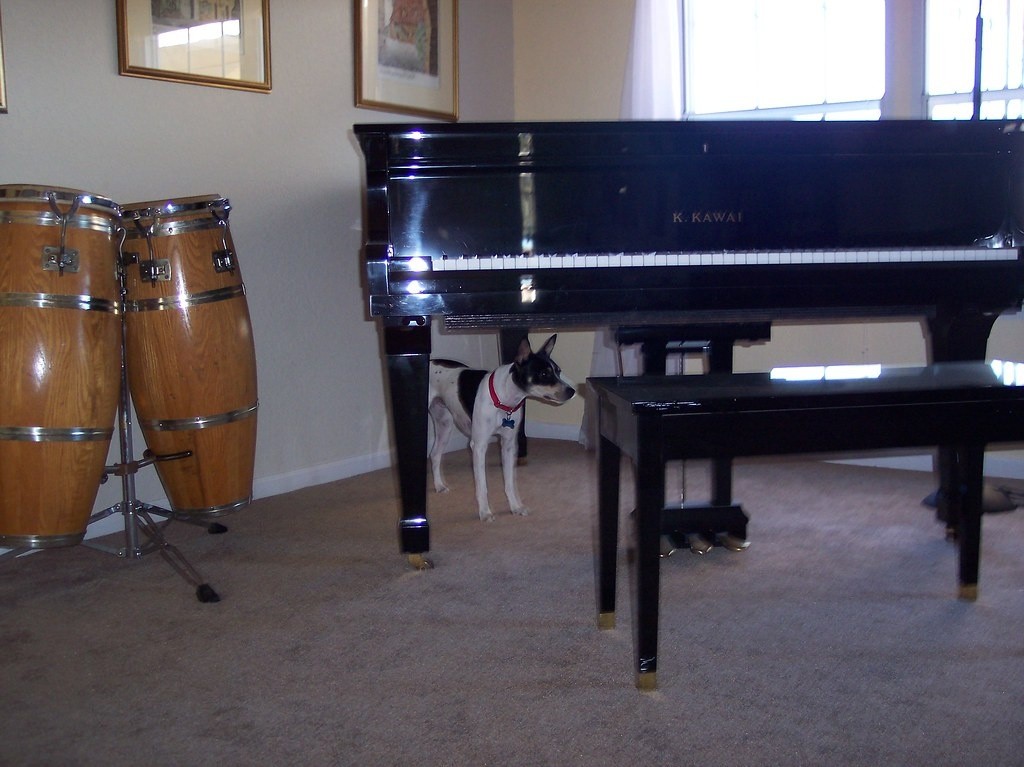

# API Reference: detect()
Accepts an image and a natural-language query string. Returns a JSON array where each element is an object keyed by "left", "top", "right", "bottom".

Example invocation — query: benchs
[{"left": 582, "top": 361, "right": 1024, "bottom": 691}]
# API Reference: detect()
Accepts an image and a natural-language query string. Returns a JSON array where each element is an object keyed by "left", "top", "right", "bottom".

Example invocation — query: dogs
[{"left": 428, "top": 333, "right": 576, "bottom": 525}]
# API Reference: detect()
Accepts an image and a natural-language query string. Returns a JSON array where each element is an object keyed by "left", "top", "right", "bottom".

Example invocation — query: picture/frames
[
  {"left": 354, "top": 0, "right": 459, "bottom": 121},
  {"left": 115, "top": 0, "right": 273, "bottom": 94}
]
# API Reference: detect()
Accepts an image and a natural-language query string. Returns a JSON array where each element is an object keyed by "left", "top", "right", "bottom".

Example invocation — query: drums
[
  {"left": 0, "top": 182, "right": 120, "bottom": 550},
  {"left": 114, "top": 194, "right": 259, "bottom": 520}
]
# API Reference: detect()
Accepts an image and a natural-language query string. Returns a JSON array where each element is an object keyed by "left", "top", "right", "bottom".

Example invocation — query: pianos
[{"left": 351, "top": 118, "right": 1023, "bottom": 556}]
